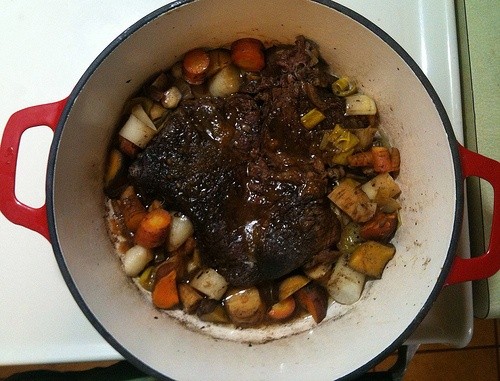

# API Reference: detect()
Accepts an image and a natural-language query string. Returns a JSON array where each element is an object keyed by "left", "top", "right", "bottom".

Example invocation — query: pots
[{"left": 1, "top": 0, "right": 500, "bottom": 381}]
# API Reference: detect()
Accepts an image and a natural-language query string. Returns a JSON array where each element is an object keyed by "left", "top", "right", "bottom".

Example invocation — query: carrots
[{"left": 123, "top": 39, "right": 391, "bottom": 314}]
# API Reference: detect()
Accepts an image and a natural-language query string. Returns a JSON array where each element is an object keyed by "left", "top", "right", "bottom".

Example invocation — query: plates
[{"left": 1, "top": 1, "right": 474, "bottom": 365}]
